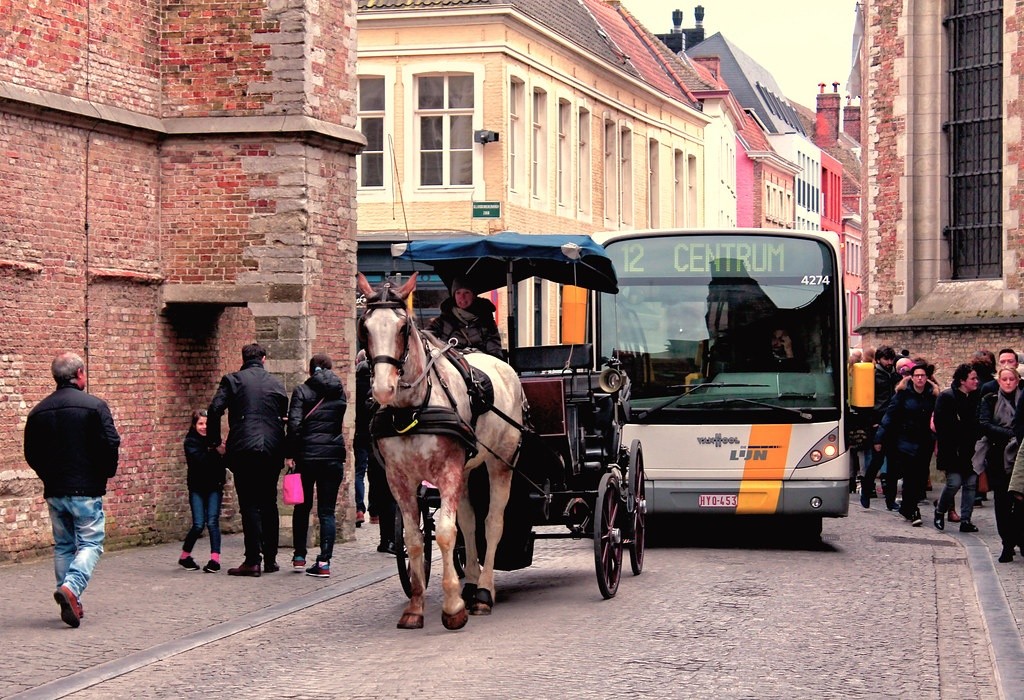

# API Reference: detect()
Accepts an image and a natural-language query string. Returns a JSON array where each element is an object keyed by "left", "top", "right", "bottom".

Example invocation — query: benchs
[
  {"left": 518, "top": 377, "right": 564, "bottom": 436},
  {"left": 514, "top": 344, "right": 594, "bottom": 401}
]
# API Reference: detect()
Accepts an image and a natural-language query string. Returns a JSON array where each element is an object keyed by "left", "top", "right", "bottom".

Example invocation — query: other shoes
[
  {"left": 860, "top": 488, "right": 871, "bottom": 508},
  {"left": 998, "top": 548, "right": 1016, "bottom": 562},
  {"left": 912, "top": 512, "right": 922, "bottom": 526},
  {"left": 377, "top": 544, "right": 387, "bottom": 551},
  {"left": 355, "top": 511, "right": 364, "bottom": 524},
  {"left": 890, "top": 503, "right": 900, "bottom": 511},
  {"left": 387, "top": 541, "right": 408, "bottom": 558},
  {"left": 370, "top": 516, "right": 379, "bottom": 523}
]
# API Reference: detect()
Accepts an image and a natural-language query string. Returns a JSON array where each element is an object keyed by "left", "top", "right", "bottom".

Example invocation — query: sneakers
[
  {"left": 306, "top": 560, "right": 331, "bottom": 577},
  {"left": 203, "top": 559, "right": 221, "bottom": 573},
  {"left": 178, "top": 556, "right": 199, "bottom": 571},
  {"left": 77, "top": 604, "right": 83, "bottom": 617},
  {"left": 55, "top": 586, "right": 81, "bottom": 628},
  {"left": 292, "top": 556, "right": 305, "bottom": 568}
]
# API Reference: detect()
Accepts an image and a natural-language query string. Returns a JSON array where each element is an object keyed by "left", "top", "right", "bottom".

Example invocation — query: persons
[
  {"left": 23, "top": 352, "right": 121, "bottom": 628},
  {"left": 762, "top": 328, "right": 812, "bottom": 372},
  {"left": 286, "top": 355, "right": 347, "bottom": 577},
  {"left": 849, "top": 345, "right": 1024, "bottom": 562},
  {"left": 177, "top": 409, "right": 226, "bottom": 573},
  {"left": 206, "top": 344, "right": 288, "bottom": 576},
  {"left": 425, "top": 276, "right": 503, "bottom": 358},
  {"left": 353, "top": 350, "right": 410, "bottom": 558}
]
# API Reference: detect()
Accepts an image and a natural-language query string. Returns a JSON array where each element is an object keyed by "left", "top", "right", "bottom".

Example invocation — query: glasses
[{"left": 913, "top": 374, "right": 926, "bottom": 377}]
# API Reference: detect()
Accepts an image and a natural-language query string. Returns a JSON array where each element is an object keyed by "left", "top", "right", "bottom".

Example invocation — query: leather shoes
[
  {"left": 228, "top": 562, "right": 261, "bottom": 577},
  {"left": 960, "top": 523, "right": 978, "bottom": 532},
  {"left": 264, "top": 562, "right": 280, "bottom": 572},
  {"left": 933, "top": 509, "right": 945, "bottom": 530}
]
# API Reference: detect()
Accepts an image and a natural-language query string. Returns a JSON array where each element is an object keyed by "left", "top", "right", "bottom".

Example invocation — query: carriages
[{"left": 359, "top": 232, "right": 646, "bottom": 629}]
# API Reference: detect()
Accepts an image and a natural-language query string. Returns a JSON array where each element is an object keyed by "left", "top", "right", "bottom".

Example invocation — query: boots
[{"left": 948, "top": 509, "right": 960, "bottom": 522}]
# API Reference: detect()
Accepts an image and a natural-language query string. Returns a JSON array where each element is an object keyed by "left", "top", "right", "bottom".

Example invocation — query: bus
[{"left": 563, "top": 228, "right": 875, "bottom": 540}]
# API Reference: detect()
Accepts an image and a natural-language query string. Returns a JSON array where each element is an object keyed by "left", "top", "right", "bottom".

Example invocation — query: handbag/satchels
[{"left": 282, "top": 468, "right": 304, "bottom": 505}]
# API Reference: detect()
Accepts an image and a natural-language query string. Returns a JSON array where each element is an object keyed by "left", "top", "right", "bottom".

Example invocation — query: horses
[{"left": 356, "top": 272, "right": 523, "bottom": 629}]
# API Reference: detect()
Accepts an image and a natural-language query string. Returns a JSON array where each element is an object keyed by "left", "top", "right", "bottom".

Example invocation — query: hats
[{"left": 451, "top": 275, "right": 476, "bottom": 297}]
